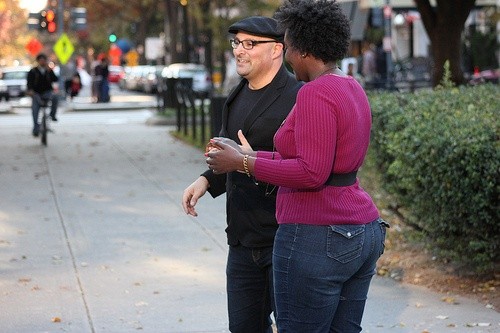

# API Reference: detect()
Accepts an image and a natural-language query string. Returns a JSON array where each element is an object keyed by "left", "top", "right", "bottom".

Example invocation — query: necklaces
[{"left": 319, "top": 66, "right": 339, "bottom": 75}]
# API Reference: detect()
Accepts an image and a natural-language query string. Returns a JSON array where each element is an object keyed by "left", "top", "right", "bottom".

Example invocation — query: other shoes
[
  {"left": 49, "top": 113, "right": 58, "bottom": 121},
  {"left": 33, "top": 128, "right": 39, "bottom": 137}
]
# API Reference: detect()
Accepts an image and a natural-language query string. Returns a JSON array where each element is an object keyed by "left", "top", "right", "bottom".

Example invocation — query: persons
[
  {"left": 204, "top": 0, "right": 389, "bottom": 333},
  {"left": 96, "top": 57, "right": 110, "bottom": 102},
  {"left": 27, "top": 54, "right": 58, "bottom": 138},
  {"left": 181, "top": 15, "right": 308, "bottom": 333}
]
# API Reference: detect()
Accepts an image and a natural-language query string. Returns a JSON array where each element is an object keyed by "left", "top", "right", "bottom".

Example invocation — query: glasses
[{"left": 229, "top": 39, "right": 278, "bottom": 50}]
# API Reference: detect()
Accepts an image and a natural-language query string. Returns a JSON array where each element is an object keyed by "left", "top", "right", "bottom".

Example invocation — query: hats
[{"left": 227, "top": 15, "right": 285, "bottom": 44}]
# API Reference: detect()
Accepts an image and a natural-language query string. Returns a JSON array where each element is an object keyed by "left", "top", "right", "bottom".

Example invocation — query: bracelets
[{"left": 242, "top": 155, "right": 251, "bottom": 178}]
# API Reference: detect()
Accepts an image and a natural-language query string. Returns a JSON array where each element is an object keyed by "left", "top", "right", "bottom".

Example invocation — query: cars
[
  {"left": 108, "top": 65, "right": 123, "bottom": 81},
  {"left": 0, "top": 66, "right": 32, "bottom": 98},
  {"left": 118, "top": 63, "right": 212, "bottom": 96}
]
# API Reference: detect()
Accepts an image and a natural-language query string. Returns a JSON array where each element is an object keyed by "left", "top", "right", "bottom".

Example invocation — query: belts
[{"left": 326, "top": 171, "right": 357, "bottom": 186}]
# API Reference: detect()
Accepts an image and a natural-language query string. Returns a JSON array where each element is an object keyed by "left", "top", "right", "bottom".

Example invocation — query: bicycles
[{"left": 28, "top": 90, "right": 58, "bottom": 146}]
